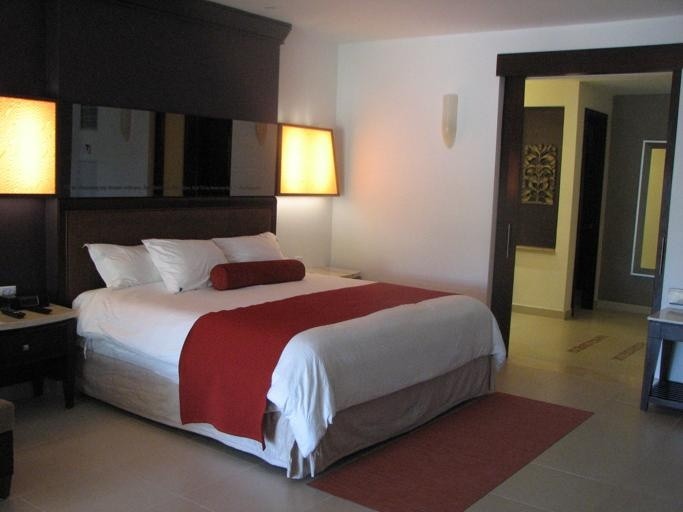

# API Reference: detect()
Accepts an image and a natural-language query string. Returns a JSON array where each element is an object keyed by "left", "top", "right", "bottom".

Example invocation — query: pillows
[
  {"left": 142, "top": 239, "right": 229, "bottom": 294},
  {"left": 211, "top": 232, "right": 289, "bottom": 263},
  {"left": 210, "top": 259, "right": 305, "bottom": 290},
  {"left": 82, "top": 242, "right": 164, "bottom": 290}
]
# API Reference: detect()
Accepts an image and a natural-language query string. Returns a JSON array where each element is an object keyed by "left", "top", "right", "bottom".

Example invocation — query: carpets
[{"left": 306, "top": 391, "right": 595, "bottom": 512}]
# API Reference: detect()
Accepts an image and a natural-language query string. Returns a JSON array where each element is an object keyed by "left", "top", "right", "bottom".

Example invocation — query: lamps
[
  {"left": 0, "top": 96, "right": 56, "bottom": 195},
  {"left": 275, "top": 123, "right": 341, "bottom": 196}
]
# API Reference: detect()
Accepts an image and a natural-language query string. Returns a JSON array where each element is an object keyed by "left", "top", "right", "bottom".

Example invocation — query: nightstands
[
  {"left": 306, "top": 266, "right": 362, "bottom": 280},
  {"left": 0, "top": 303, "right": 79, "bottom": 409}
]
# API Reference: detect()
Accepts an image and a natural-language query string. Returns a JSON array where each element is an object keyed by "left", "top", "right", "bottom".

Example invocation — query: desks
[{"left": 640, "top": 307, "right": 683, "bottom": 412}]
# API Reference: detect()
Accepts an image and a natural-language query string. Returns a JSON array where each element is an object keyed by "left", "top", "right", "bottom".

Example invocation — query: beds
[{"left": 61, "top": 196, "right": 507, "bottom": 481}]
